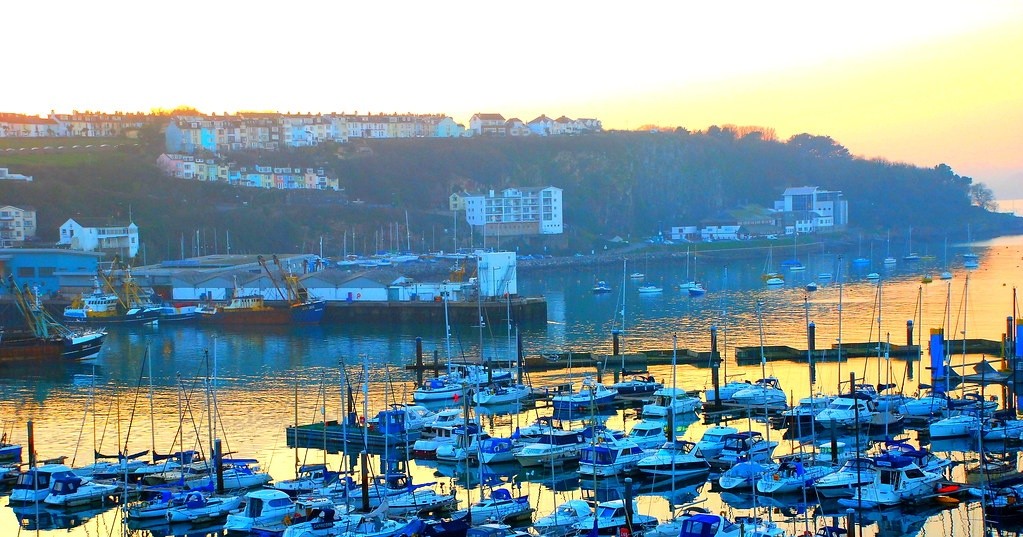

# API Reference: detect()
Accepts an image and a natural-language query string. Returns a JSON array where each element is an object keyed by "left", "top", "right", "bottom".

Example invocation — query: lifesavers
[
  {"left": 720, "top": 511, "right": 726, "bottom": 517},
  {"left": 141, "top": 502, "right": 148, "bottom": 508},
  {"left": 598, "top": 437, "right": 603, "bottom": 442}
]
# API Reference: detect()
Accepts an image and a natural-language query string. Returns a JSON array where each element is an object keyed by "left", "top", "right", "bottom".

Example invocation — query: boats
[
  {"left": 767, "top": 277, "right": 785, "bottom": 284},
  {"left": 331, "top": 209, "right": 477, "bottom": 267},
  {"left": 631, "top": 273, "right": 644, "bottom": 278},
  {"left": 789, "top": 266, "right": 805, "bottom": 270},
  {"left": 59, "top": 254, "right": 159, "bottom": 323},
  {"left": 940, "top": 271, "right": 952, "bottom": 279},
  {"left": 963, "top": 254, "right": 979, "bottom": 267},
  {"left": 638, "top": 286, "right": 662, "bottom": 293},
  {"left": 0, "top": 359, "right": 1023, "bottom": 537},
  {"left": 1, "top": 262, "right": 108, "bottom": 362},
  {"left": 884, "top": 258, "right": 896, "bottom": 263},
  {"left": 680, "top": 282, "right": 706, "bottom": 294},
  {"left": 904, "top": 252, "right": 919, "bottom": 258},
  {"left": 152, "top": 304, "right": 213, "bottom": 318},
  {"left": 192, "top": 252, "right": 325, "bottom": 324},
  {"left": 866, "top": 273, "right": 880, "bottom": 279},
  {"left": 806, "top": 281, "right": 816, "bottom": 291},
  {"left": 922, "top": 274, "right": 932, "bottom": 282},
  {"left": 594, "top": 280, "right": 610, "bottom": 292},
  {"left": 819, "top": 276, "right": 832, "bottom": 278}
]
